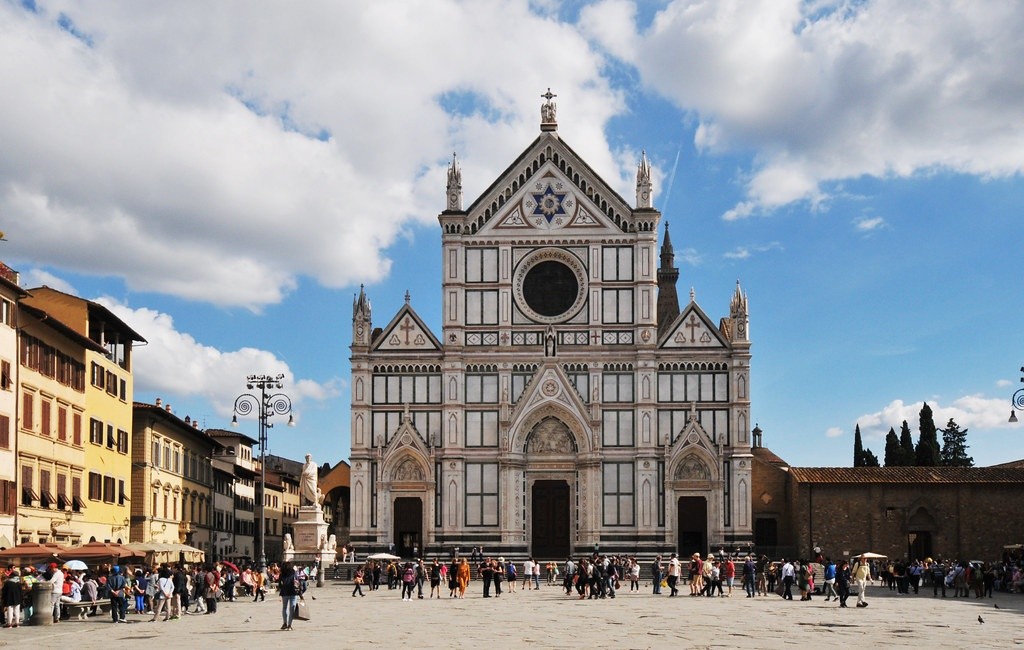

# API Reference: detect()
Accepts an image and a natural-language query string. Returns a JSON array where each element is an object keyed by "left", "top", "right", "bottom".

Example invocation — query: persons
[
  {"left": 1, "top": 541, "right": 1024, "bottom": 629},
  {"left": 299, "top": 454, "right": 318, "bottom": 505}
]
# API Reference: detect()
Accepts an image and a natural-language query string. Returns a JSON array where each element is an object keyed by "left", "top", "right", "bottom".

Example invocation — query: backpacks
[
  {"left": 592, "top": 564, "right": 601, "bottom": 580},
  {"left": 263, "top": 577, "right": 270, "bottom": 586}
]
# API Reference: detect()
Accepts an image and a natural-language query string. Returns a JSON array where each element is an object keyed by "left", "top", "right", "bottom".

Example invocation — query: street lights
[{"left": 231, "top": 374, "right": 295, "bottom": 572}]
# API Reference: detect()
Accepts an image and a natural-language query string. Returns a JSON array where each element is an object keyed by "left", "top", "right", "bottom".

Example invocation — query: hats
[
  {"left": 98, "top": 576, "right": 107, "bottom": 584},
  {"left": 707, "top": 554, "right": 714, "bottom": 559},
  {"left": 497, "top": 557, "right": 505, "bottom": 562},
  {"left": 111, "top": 565, "right": 120, "bottom": 575},
  {"left": 48, "top": 563, "right": 57, "bottom": 568},
  {"left": 21, "top": 567, "right": 32, "bottom": 573},
  {"left": 692, "top": 552, "right": 700, "bottom": 557}
]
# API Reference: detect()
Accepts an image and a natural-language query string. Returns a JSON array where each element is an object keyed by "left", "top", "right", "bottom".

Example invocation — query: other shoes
[{"left": 0, "top": 616, "right": 29, "bottom": 628}]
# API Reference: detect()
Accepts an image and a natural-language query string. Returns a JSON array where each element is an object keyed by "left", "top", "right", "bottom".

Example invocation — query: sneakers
[
  {"left": 280, "top": 623, "right": 293, "bottom": 631},
  {"left": 53, "top": 591, "right": 270, "bottom": 623},
  {"left": 270, "top": 570, "right": 1024, "bottom": 608}
]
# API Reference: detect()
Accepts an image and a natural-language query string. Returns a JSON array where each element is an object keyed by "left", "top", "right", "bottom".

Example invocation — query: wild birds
[
  {"left": 244, "top": 616, "right": 253, "bottom": 624},
  {"left": 978, "top": 615, "right": 986, "bottom": 624},
  {"left": 312, "top": 595, "right": 317, "bottom": 600},
  {"left": 993, "top": 603, "right": 1001, "bottom": 610}
]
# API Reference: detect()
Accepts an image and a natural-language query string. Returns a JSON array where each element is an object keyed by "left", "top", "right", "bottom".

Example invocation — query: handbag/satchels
[{"left": 296, "top": 602, "right": 311, "bottom": 621}]
